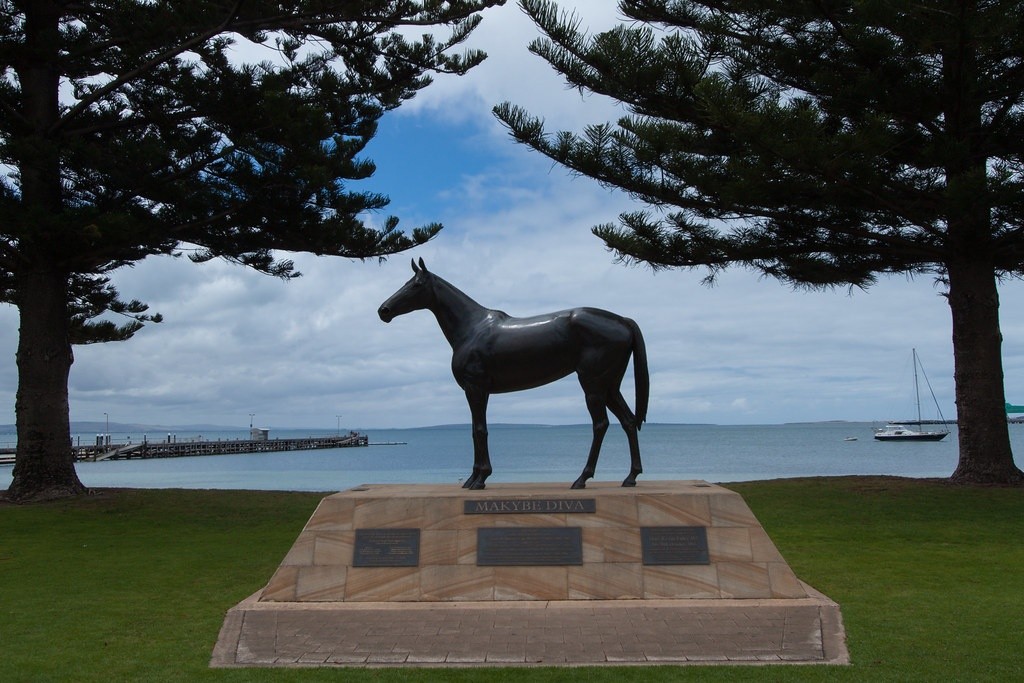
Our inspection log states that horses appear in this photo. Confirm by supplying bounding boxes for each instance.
[378,257,649,491]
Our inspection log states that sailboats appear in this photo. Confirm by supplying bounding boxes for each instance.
[872,347,949,442]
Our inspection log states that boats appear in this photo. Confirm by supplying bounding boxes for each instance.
[842,434,858,442]
